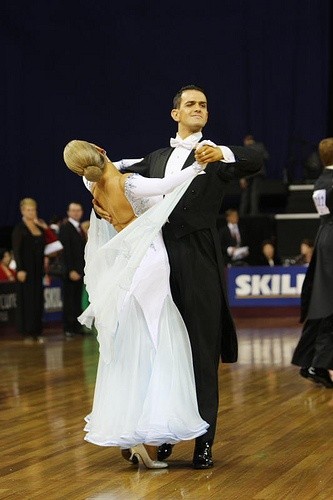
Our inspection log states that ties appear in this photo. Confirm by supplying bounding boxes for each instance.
[79,225,86,239]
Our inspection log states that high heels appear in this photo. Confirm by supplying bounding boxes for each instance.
[120,448,138,463]
[128,444,168,469]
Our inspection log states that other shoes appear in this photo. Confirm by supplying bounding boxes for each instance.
[308,367,333,388]
[300,368,318,383]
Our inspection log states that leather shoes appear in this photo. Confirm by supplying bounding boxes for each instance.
[192,442,213,468]
[157,443,172,461]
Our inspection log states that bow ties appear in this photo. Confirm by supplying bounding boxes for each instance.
[170,137,195,150]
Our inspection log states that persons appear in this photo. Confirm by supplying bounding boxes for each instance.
[290,138,333,389]
[12,198,49,344]
[63,140,218,469]
[0,134,315,282]
[59,201,90,337]
[91,85,265,469]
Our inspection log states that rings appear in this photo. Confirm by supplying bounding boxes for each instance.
[202,152,205,155]
